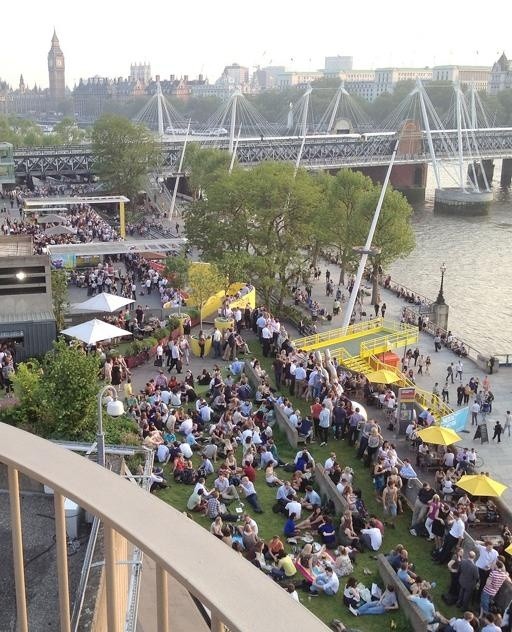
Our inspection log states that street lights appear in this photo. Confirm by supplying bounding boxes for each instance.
[435,262,448,306]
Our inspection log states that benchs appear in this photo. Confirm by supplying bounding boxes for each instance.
[374,550,438,632]
[273,401,306,449]
[243,356,262,393]
[312,462,359,521]
[231,330,246,358]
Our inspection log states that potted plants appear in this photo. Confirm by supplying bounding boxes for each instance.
[211,254,250,336]
[184,261,227,358]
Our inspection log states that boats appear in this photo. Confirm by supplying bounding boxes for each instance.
[34,120,232,137]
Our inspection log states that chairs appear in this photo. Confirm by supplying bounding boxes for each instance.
[368,387,512,578]
